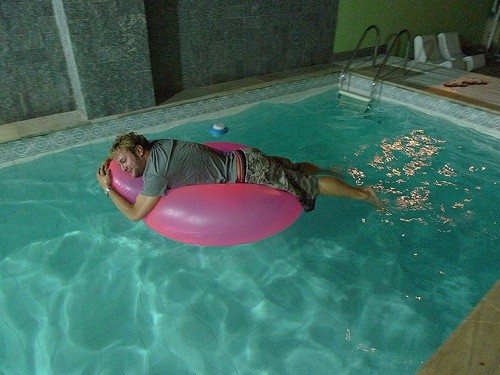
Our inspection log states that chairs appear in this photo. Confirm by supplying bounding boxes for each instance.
[414,34,467,72]
[437,32,485,72]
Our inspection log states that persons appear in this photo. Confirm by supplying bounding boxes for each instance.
[95,132,389,223]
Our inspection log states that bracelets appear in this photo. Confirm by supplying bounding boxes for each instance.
[104,186,112,197]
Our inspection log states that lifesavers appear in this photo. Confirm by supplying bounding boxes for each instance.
[106,141,304,244]
